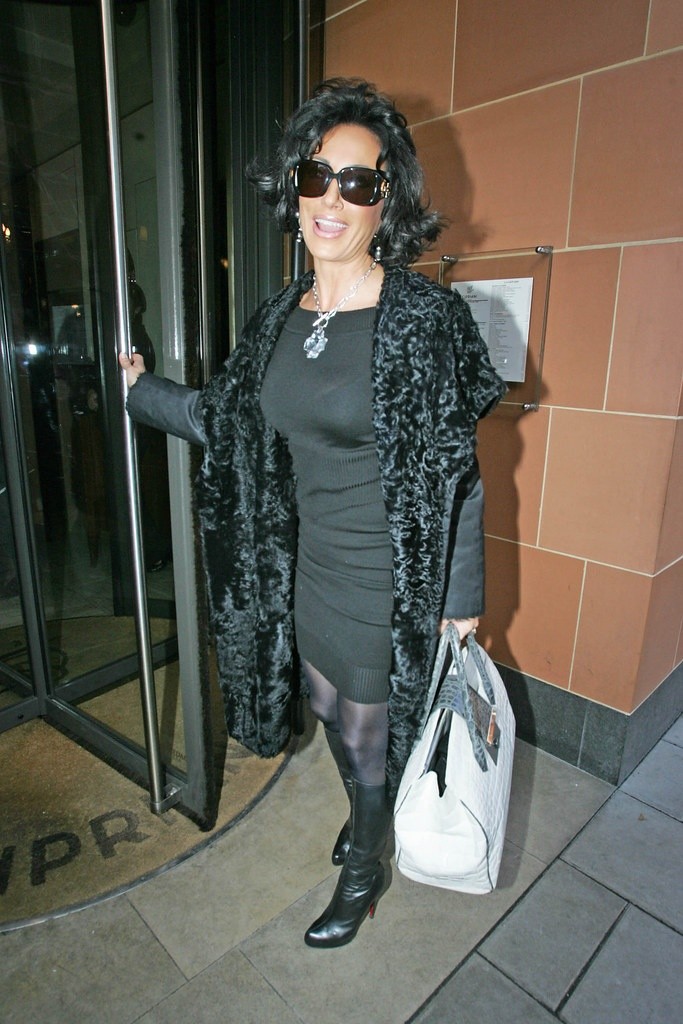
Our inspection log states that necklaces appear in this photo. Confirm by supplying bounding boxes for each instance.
[303,262,376,360]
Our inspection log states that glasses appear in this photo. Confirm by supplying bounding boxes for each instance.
[290,158,393,206]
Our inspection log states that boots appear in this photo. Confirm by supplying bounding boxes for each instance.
[323,725,357,867]
[304,775,389,949]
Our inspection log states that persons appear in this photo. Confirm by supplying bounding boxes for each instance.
[117,77,504,948]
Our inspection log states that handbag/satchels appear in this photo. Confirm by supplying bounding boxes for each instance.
[392,621,516,895]
